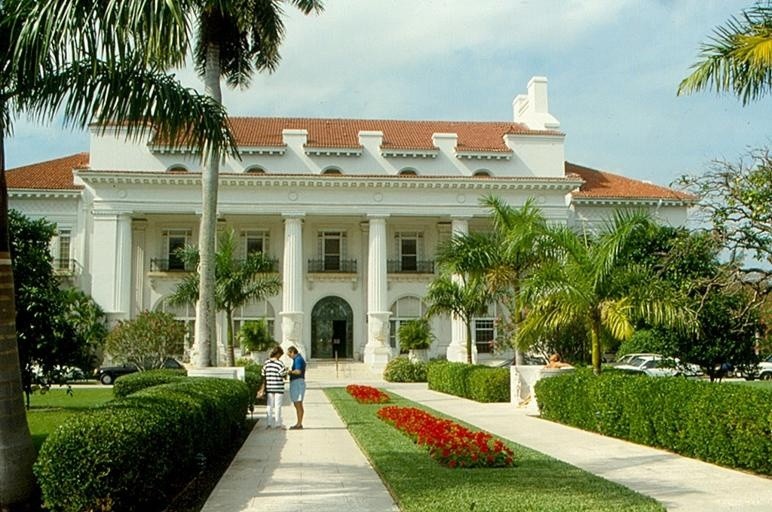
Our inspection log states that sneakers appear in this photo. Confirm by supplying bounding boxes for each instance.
[290,425,303,430]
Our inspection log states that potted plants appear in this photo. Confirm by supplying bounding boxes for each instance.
[238,315,281,365]
[398,319,437,365]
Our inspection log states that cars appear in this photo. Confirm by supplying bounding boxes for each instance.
[604,350,701,380]
[703,350,755,378]
[29,359,86,385]
[91,355,188,386]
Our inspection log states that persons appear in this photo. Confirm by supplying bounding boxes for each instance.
[287,346,306,429]
[517,353,570,407]
[261,346,289,431]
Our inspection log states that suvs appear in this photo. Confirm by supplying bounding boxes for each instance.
[740,353,772,383]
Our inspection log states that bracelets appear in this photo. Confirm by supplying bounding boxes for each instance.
[291,372,292,375]
